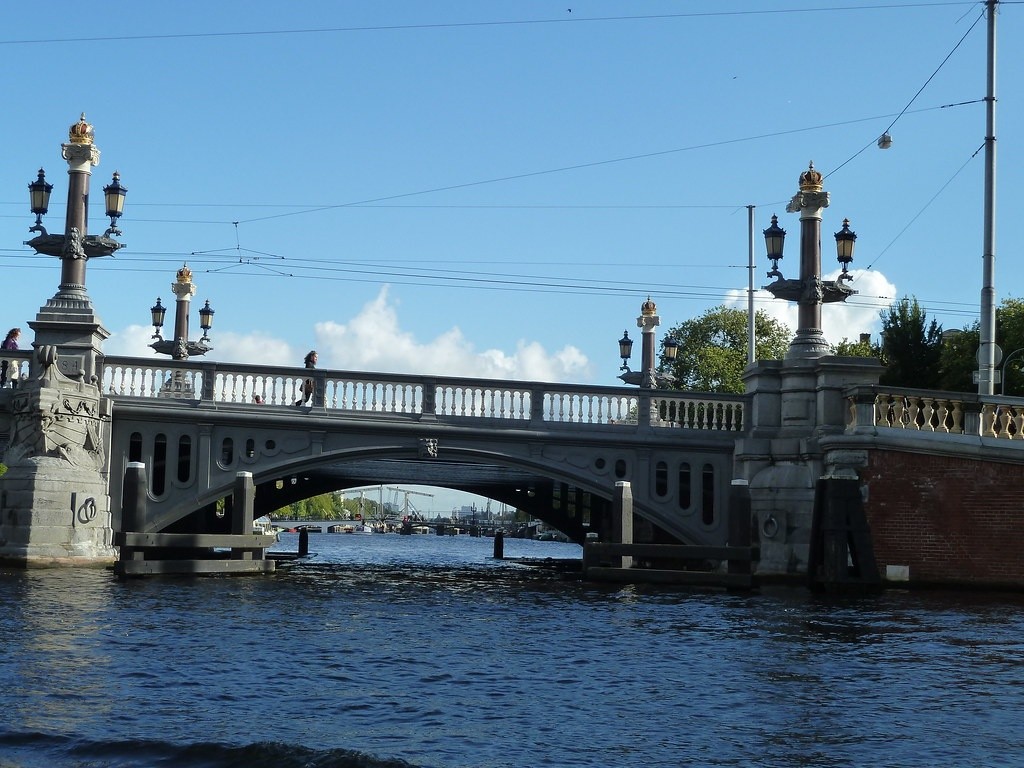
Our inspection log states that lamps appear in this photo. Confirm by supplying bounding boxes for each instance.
[762,214,787,271]
[150,296,167,335]
[103,169,128,231]
[878,134,892,148]
[28,167,54,226]
[834,219,857,275]
[198,299,215,338]
[664,336,679,365]
[618,330,633,367]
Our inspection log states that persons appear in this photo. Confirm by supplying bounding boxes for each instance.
[290,351,318,407]
[0,328,20,388]
[255,395,262,406]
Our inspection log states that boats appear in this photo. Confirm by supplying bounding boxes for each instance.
[253,516,280,542]
[327,524,354,533]
[294,525,322,533]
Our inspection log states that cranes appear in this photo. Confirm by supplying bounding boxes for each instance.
[386,487,434,505]
[341,487,383,514]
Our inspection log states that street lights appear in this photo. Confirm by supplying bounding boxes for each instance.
[147,262,215,376]
[616,294,678,406]
[761,160,859,336]
[22,113,127,290]
